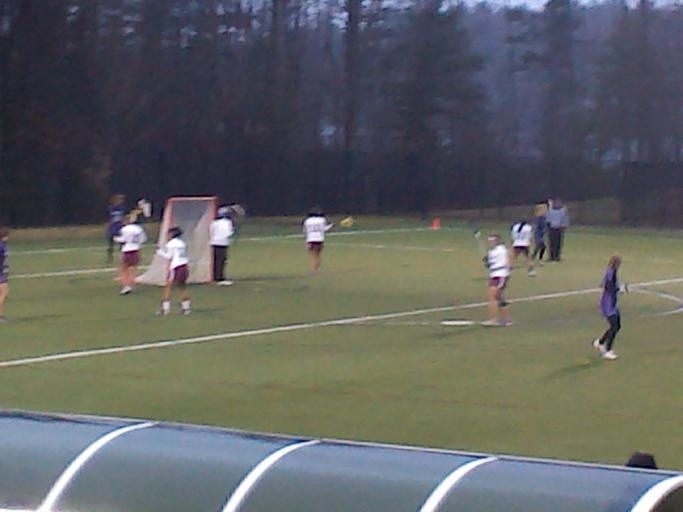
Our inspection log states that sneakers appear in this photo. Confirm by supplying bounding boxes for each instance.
[214,278,236,287]
[119,285,134,296]
[592,338,620,361]
[478,316,517,330]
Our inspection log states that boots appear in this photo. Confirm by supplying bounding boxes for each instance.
[177,297,193,317]
[153,298,173,317]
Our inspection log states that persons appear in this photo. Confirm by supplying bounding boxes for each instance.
[591,255,623,360]
[301,210,335,271]
[105,193,244,317]
[0,230,11,322]
[481,196,569,327]
